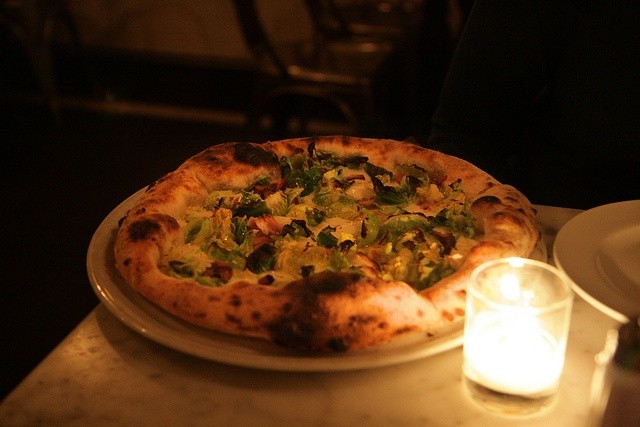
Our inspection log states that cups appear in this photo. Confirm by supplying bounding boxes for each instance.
[463,258,573,421]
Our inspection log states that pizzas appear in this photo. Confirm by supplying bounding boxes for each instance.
[112,132,540,355]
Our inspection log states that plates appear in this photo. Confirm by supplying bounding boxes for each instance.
[553,200,640,323]
[88,180,547,374]
